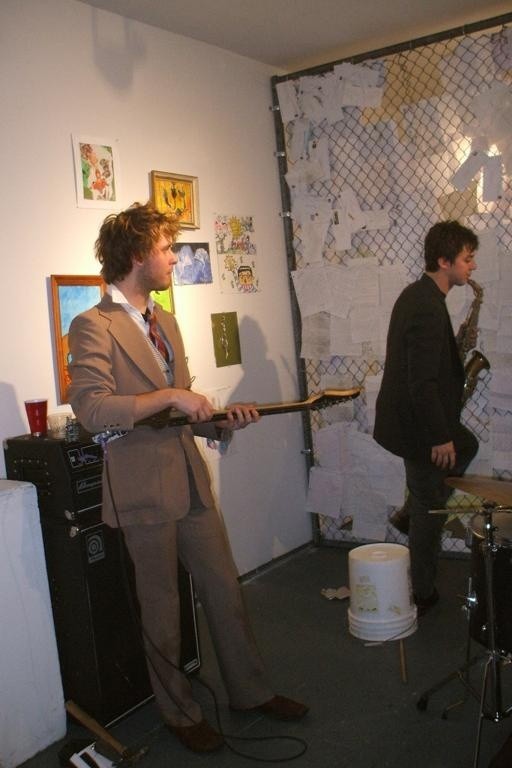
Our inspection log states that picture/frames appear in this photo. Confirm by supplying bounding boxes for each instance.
[150,169,199,231]
[50,273,107,401]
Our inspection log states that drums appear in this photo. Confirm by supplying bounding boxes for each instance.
[468,513,512,662]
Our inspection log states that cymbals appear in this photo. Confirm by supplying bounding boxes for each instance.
[445,476,511,506]
[427,508,512,515]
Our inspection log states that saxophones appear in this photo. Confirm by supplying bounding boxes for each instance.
[456,277,489,411]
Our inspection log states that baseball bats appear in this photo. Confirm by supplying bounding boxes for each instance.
[64,690,131,758]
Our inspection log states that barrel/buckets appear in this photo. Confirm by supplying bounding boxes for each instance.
[347,541,419,647]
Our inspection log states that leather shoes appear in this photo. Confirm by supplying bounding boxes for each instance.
[164,718,225,753]
[412,587,438,606]
[388,509,409,535]
[231,694,311,719]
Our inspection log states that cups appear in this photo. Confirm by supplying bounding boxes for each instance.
[24,399,48,437]
[48,413,67,438]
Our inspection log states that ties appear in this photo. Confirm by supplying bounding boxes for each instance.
[145,313,168,362]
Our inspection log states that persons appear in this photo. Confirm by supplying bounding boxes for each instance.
[67,203,312,755]
[371,219,479,617]
